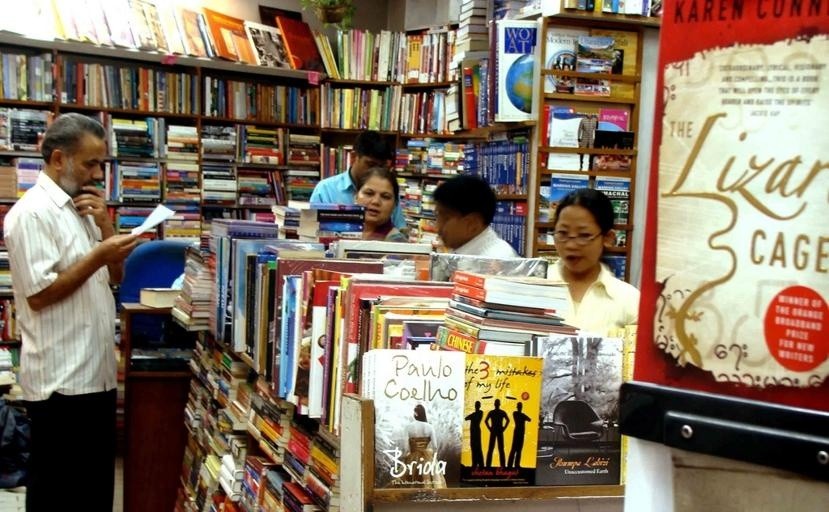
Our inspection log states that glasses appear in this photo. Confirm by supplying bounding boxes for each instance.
[551,231,602,246]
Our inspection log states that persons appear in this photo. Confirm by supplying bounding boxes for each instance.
[309,129,408,236]
[353,169,409,246]
[3,112,143,512]
[543,187,643,340]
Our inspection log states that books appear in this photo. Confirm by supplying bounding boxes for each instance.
[362,349,466,490]
[429,269,580,355]
[544,28,637,99]
[320,84,444,134]
[446,0,537,133]
[140,288,181,308]
[564,0,661,18]
[1,0,329,75]
[320,144,355,182]
[397,177,527,262]
[1,44,326,126]
[3,108,53,217]
[536,182,630,282]
[94,113,319,248]
[171,429,341,512]
[171,201,455,435]
[312,28,454,82]
[0,246,21,401]
[397,137,530,195]
[525,337,619,486]
[540,105,631,172]
[460,357,543,488]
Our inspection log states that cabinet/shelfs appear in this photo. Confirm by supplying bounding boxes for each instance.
[1,3,659,511]
